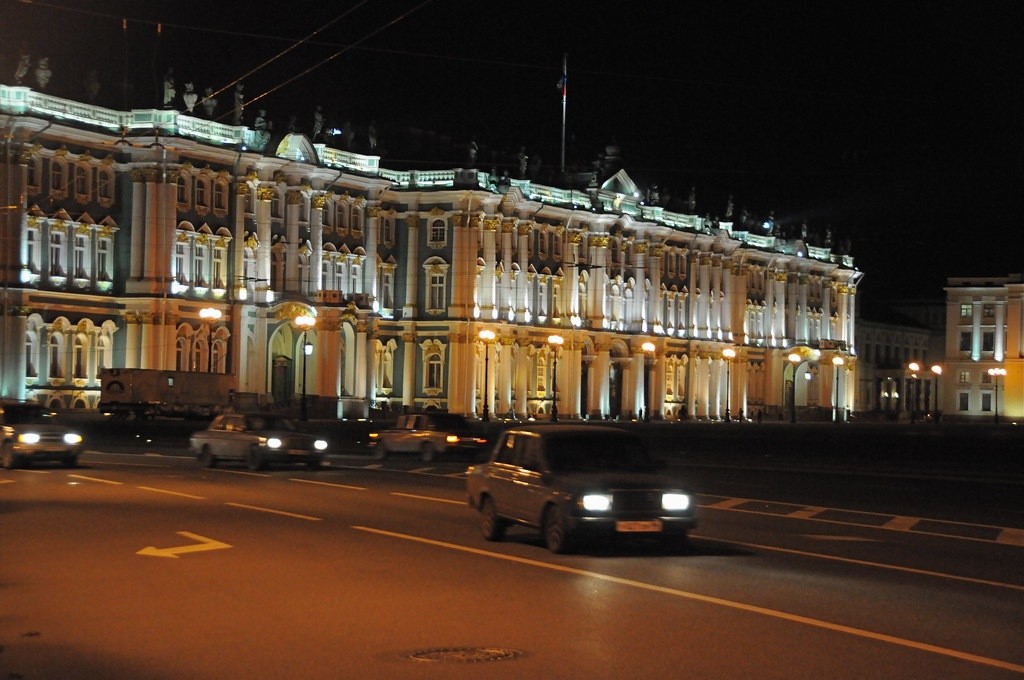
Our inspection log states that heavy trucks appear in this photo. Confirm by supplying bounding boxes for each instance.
[97,364,268,428]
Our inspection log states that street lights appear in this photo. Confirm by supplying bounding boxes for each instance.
[988,368,1007,423]
[932,365,943,422]
[909,362,921,425]
[721,348,736,424]
[295,316,316,418]
[641,342,656,421]
[479,329,495,422]
[831,357,846,423]
[786,354,802,422]
[549,334,564,419]
[200,307,220,374]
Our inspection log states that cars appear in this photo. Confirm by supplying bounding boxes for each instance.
[0,396,85,469]
[187,413,332,471]
[463,424,701,556]
[369,411,491,464]
